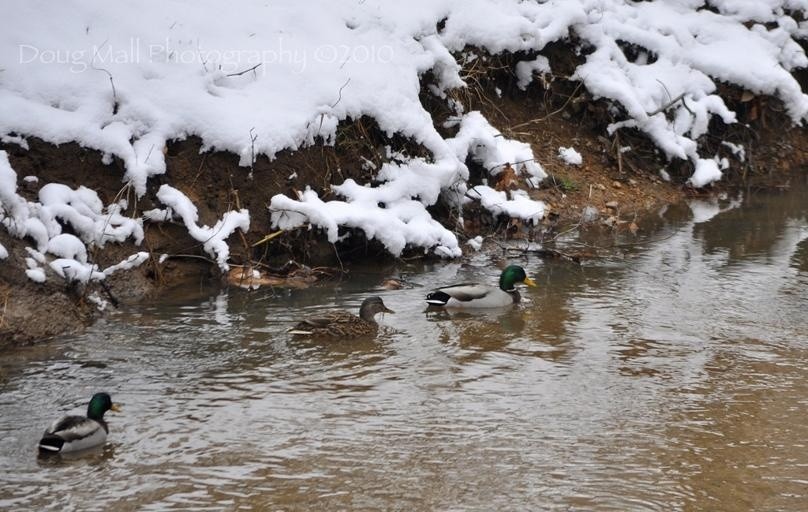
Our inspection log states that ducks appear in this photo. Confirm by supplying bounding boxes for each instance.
[38,393,122,455]
[425,265,537,306]
[285,297,395,338]
